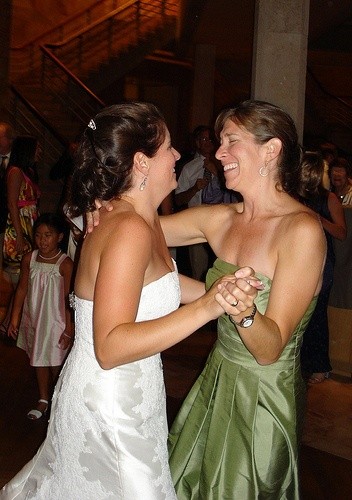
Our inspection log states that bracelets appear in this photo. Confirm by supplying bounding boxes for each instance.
[64,332,71,338]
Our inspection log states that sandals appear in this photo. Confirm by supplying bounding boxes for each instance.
[26,399,49,421]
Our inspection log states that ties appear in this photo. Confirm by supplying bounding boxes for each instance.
[203,169,213,182]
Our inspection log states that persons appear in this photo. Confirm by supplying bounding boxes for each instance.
[0,102,261,500]
[327,157,352,377]
[50,136,83,215]
[0,123,16,180]
[0,135,42,340]
[320,144,337,189]
[289,153,346,383]
[86,99,327,500]
[174,125,243,209]
[7,213,76,420]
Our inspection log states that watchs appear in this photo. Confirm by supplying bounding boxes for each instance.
[230,304,257,328]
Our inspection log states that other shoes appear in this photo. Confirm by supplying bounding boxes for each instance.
[308,372,330,384]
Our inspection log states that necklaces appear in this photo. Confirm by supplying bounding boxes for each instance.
[39,249,62,260]
